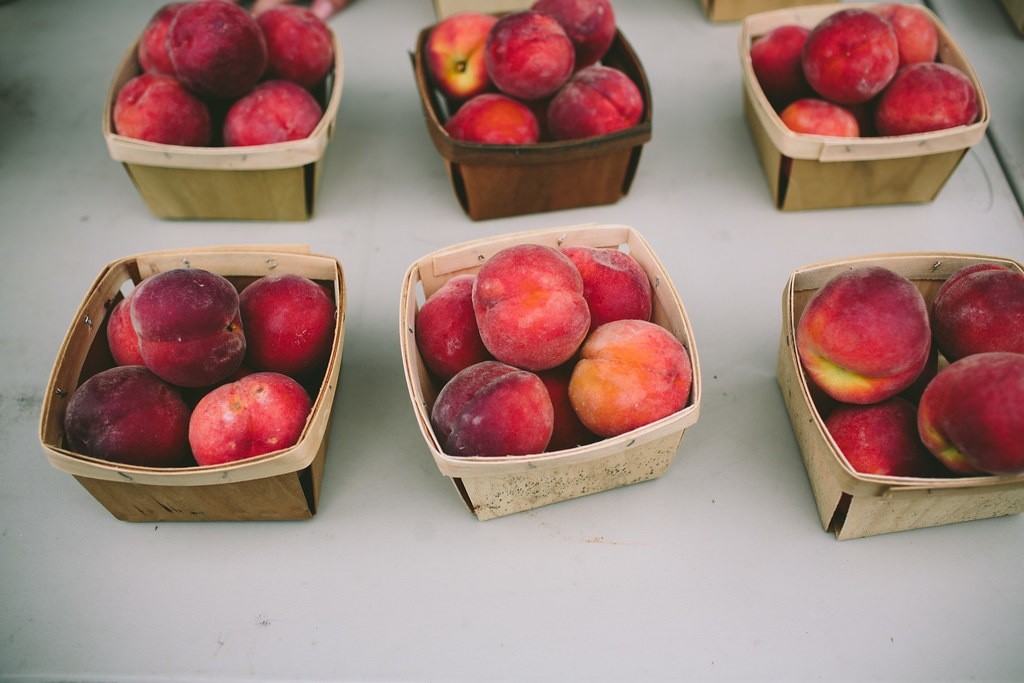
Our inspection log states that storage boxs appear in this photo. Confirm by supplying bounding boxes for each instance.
[37,242,347,524]
[101,0,343,222]
[398,222,703,524]
[736,0,991,215]
[776,251,1024,541]
[407,6,655,225]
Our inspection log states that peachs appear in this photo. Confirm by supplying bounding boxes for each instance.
[62,1,1024,485]
